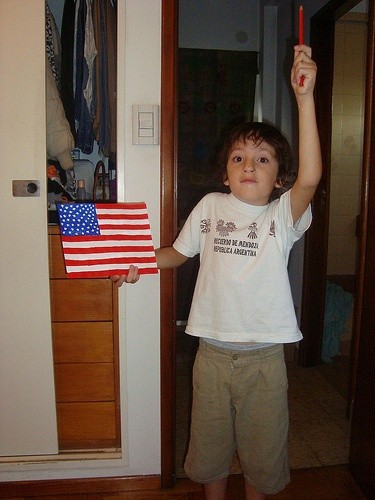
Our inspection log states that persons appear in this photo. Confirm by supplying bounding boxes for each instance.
[111,44,322,500]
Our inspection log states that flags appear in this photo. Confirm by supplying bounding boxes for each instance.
[57,203,159,278]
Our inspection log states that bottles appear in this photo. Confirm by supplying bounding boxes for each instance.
[77,180,86,200]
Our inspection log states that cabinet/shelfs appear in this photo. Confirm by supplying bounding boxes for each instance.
[48,226,121,449]
